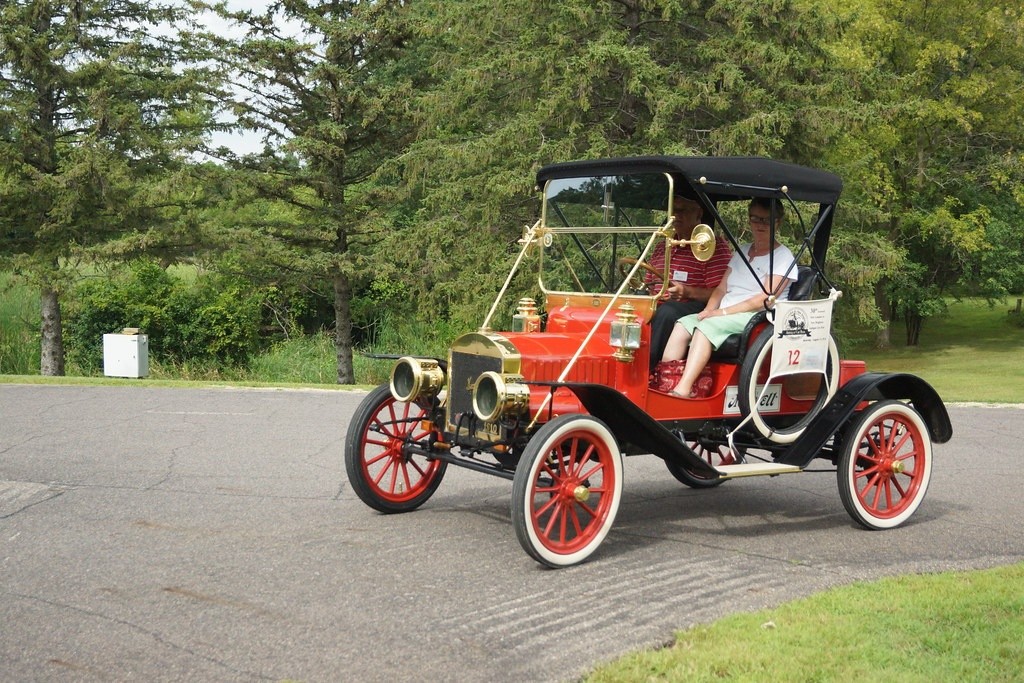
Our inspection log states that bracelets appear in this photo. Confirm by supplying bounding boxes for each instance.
[722,308,727,316]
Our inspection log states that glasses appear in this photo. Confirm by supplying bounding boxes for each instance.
[750,214,778,224]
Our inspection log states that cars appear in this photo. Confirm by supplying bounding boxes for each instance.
[346,154,954,571]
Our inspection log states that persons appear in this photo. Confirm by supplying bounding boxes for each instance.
[643,188,800,399]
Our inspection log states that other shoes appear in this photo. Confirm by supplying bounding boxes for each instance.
[668,390,690,401]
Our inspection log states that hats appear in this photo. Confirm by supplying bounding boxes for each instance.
[661,186,703,205]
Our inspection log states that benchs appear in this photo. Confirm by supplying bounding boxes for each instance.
[686,264,818,363]
